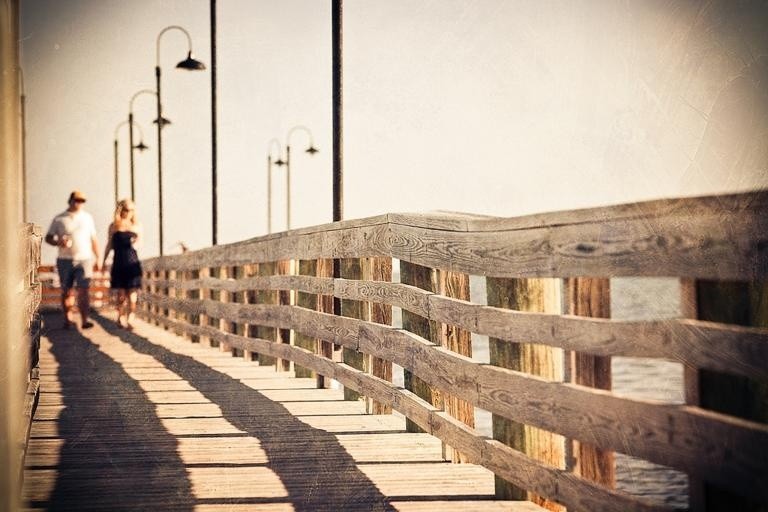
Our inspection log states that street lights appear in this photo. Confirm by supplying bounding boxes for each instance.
[264,124,318,231]
[154,23,207,260]
[113,89,169,212]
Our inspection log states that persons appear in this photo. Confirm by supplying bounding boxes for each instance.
[101,200,143,330]
[45,192,100,329]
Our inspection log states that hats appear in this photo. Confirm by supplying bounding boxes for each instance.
[70,192,85,202]
